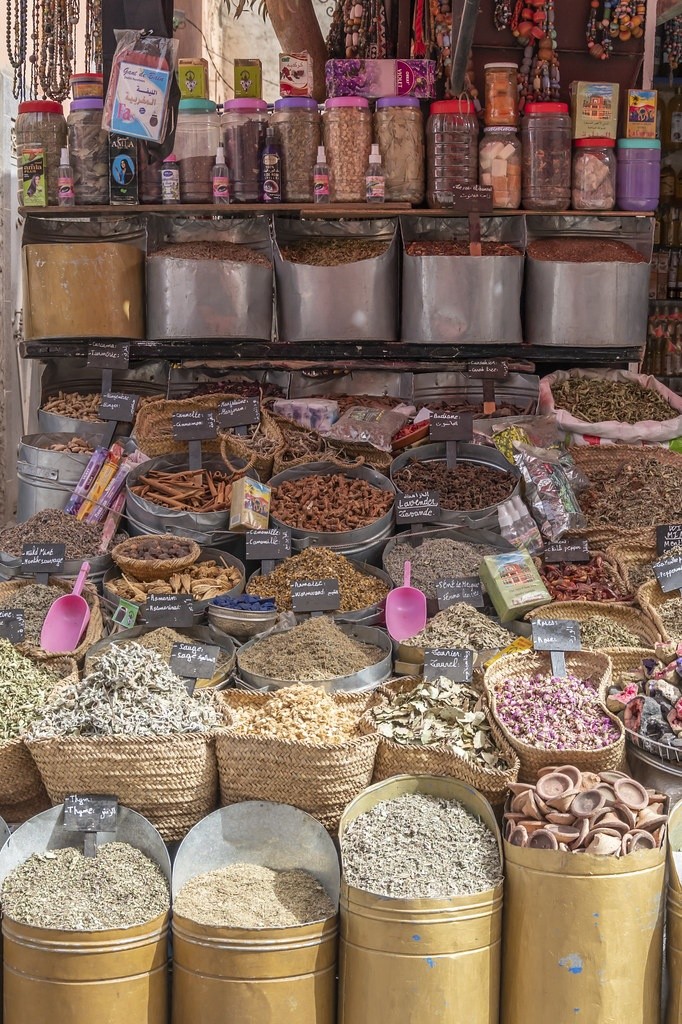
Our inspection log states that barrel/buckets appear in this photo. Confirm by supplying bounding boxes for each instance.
[0,216,656,699]
[0,773,681,1024]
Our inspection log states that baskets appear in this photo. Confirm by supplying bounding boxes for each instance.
[1,394,682,871]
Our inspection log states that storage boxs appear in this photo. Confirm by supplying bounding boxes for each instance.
[278,50,316,98]
[272,215,399,342]
[401,213,527,344]
[528,215,657,348]
[324,57,436,99]
[568,79,620,141]
[177,57,209,100]
[19,212,145,341]
[479,546,552,625]
[622,88,658,140]
[147,213,272,342]
[22,147,48,208]
[107,131,140,206]
[233,58,264,99]
[229,476,271,533]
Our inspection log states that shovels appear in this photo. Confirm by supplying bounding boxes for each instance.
[41,562,91,650]
[385,561,427,642]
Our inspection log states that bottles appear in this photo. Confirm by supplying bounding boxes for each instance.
[211,142,231,205]
[373,96,424,205]
[425,100,478,209]
[273,97,322,203]
[365,144,385,203]
[574,138,617,212]
[311,145,331,204]
[617,140,661,212]
[642,24,681,396]
[56,146,75,207]
[261,127,283,204]
[68,73,103,99]
[322,98,373,202]
[478,126,523,210]
[15,101,67,206]
[160,155,181,204]
[483,62,517,128]
[170,98,220,203]
[521,102,573,211]
[67,99,111,205]
[220,98,268,202]
